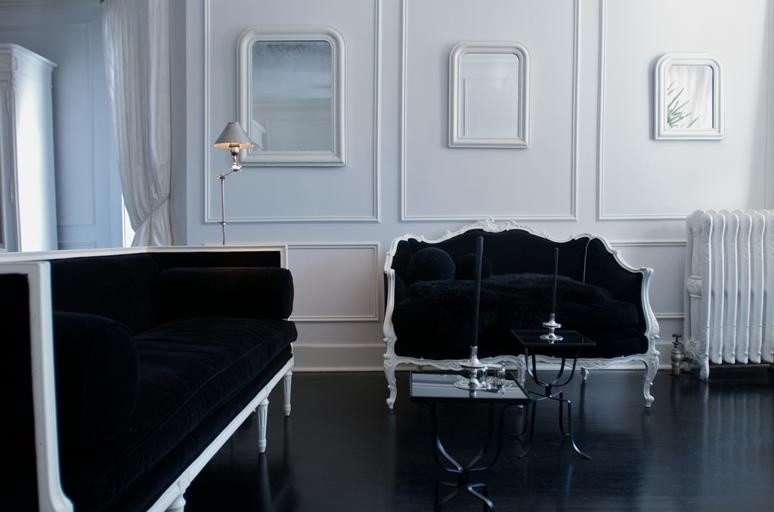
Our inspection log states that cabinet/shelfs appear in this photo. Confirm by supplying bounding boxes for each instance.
[0,43,59,253]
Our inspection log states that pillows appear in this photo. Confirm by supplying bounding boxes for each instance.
[400,247,456,284]
[53,310,141,421]
[152,267,294,321]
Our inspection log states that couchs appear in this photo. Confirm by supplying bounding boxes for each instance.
[392,219,661,411]
[0,245,298,512]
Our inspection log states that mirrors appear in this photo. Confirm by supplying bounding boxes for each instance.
[448,41,529,149]
[652,52,723,141]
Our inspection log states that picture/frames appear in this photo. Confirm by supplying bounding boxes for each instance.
[235,26,345,167]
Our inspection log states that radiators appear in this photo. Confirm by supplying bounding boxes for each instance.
[683,208,774,382]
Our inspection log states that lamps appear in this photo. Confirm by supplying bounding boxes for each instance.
[212,121,255,245]
